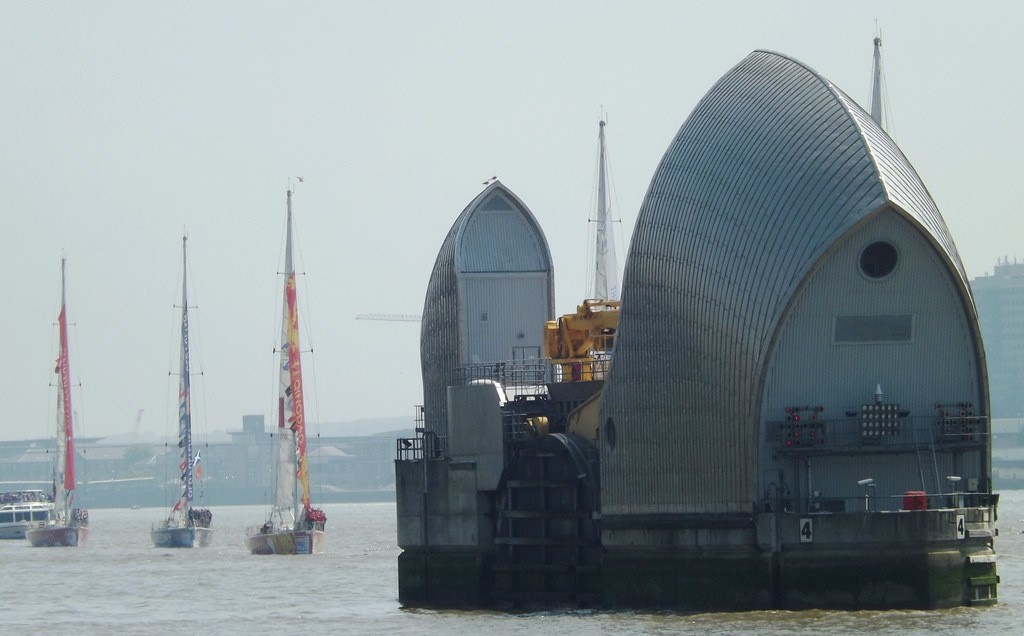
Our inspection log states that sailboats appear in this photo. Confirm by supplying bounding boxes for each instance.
[148,235,216,550]
[240,177,330,557]
[24,256,91,548]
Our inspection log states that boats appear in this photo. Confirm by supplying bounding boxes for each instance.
[389,24,1006,611]
[0,487,59,542]
